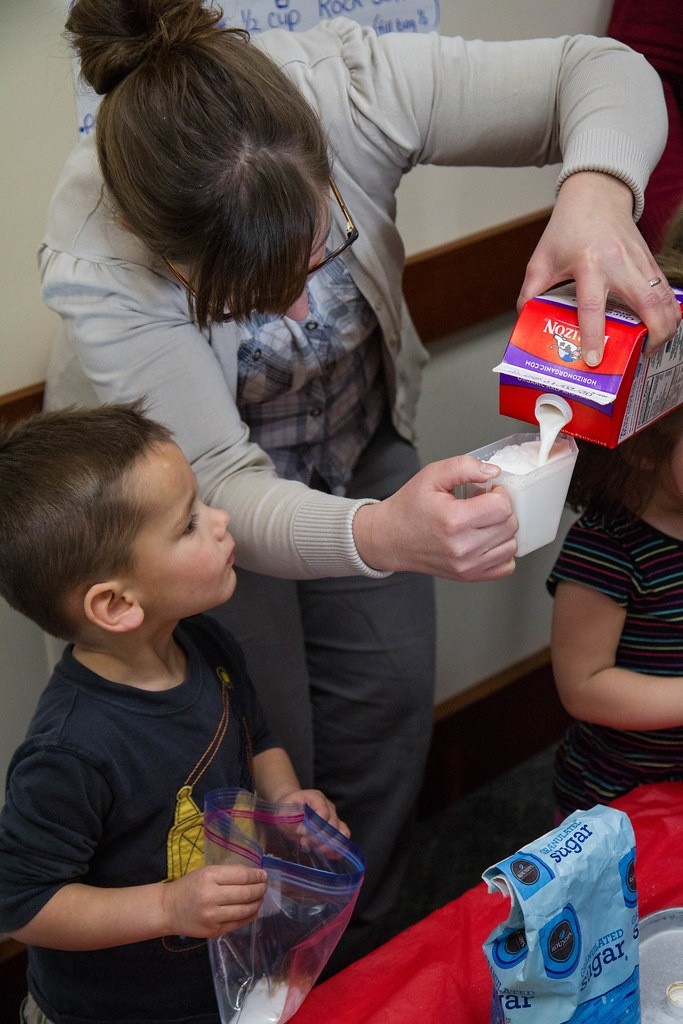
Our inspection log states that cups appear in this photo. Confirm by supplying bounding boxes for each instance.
[460,432,579,558]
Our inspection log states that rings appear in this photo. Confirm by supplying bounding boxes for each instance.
[647,274,663,288]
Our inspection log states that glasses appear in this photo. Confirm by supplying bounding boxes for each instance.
[161,175,359,324]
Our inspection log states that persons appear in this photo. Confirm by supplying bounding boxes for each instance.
[0,389,351,1023]
[545,405,683,829]
[31,0,672,960]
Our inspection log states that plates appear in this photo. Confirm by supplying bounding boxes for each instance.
[639,908,683,1024]
